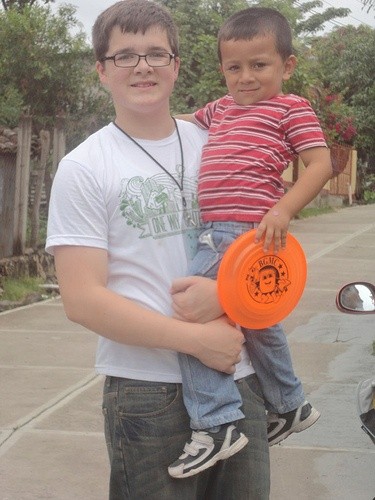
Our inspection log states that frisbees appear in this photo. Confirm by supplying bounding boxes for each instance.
[216,226,307,330]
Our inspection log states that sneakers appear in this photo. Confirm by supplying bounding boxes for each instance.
[168,423,249,479]
[266,401,321,447]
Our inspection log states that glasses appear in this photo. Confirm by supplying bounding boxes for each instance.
[98,51,175,68]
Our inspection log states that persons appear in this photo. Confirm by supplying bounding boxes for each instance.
[167,7,334,478]
[46,0,270,499]
